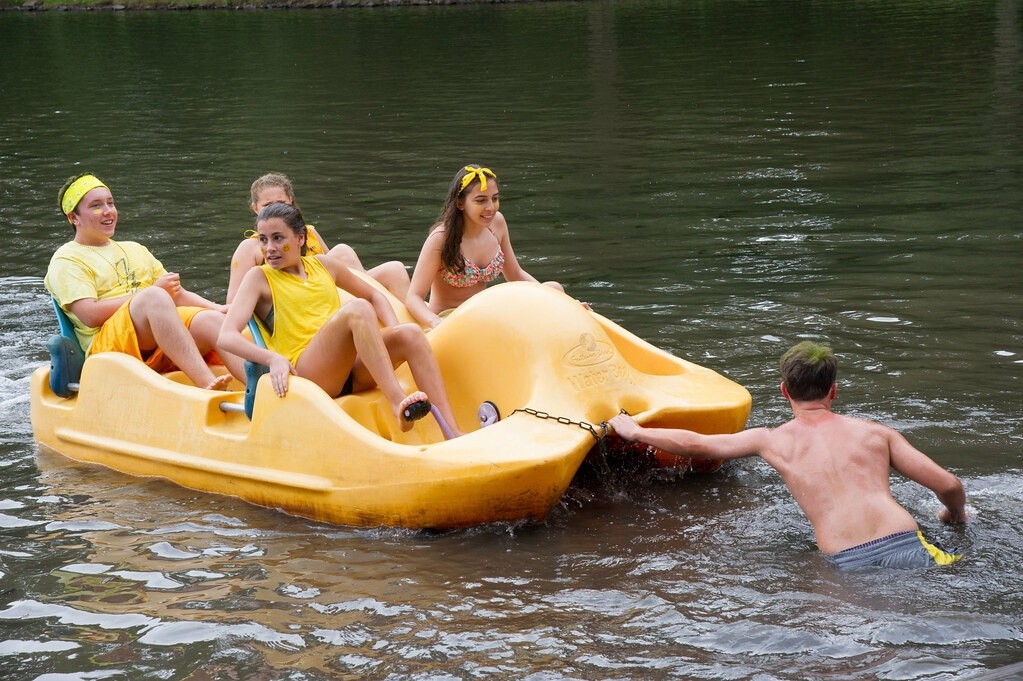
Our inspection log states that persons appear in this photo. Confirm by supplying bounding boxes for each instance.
[227,173,447,329]
[216,203,467,440]
[405,164,593,329]
[44,173,248,389]
[608,340,965,573]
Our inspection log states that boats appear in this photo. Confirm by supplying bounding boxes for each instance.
[30,281,752,528]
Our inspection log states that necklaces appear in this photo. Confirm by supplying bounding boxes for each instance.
[80,238,130,279]
